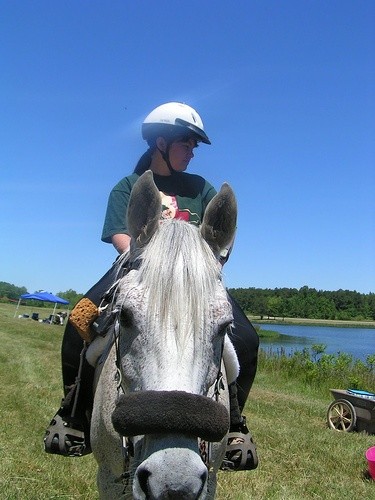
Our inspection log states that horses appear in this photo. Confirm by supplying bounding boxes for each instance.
[87,170,240,500]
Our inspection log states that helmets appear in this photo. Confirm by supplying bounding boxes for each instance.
[142,101,212,146]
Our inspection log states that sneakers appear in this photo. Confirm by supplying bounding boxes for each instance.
[229,425,252,462]
[45,414,89,447]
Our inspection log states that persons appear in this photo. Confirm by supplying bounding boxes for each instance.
[43,102,260,469]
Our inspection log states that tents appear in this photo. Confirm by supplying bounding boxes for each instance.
[14,292,69,327]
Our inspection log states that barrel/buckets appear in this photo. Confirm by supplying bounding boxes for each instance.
[366,446,375,479]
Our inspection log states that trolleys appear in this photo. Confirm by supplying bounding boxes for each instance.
[326,388,375,433]
